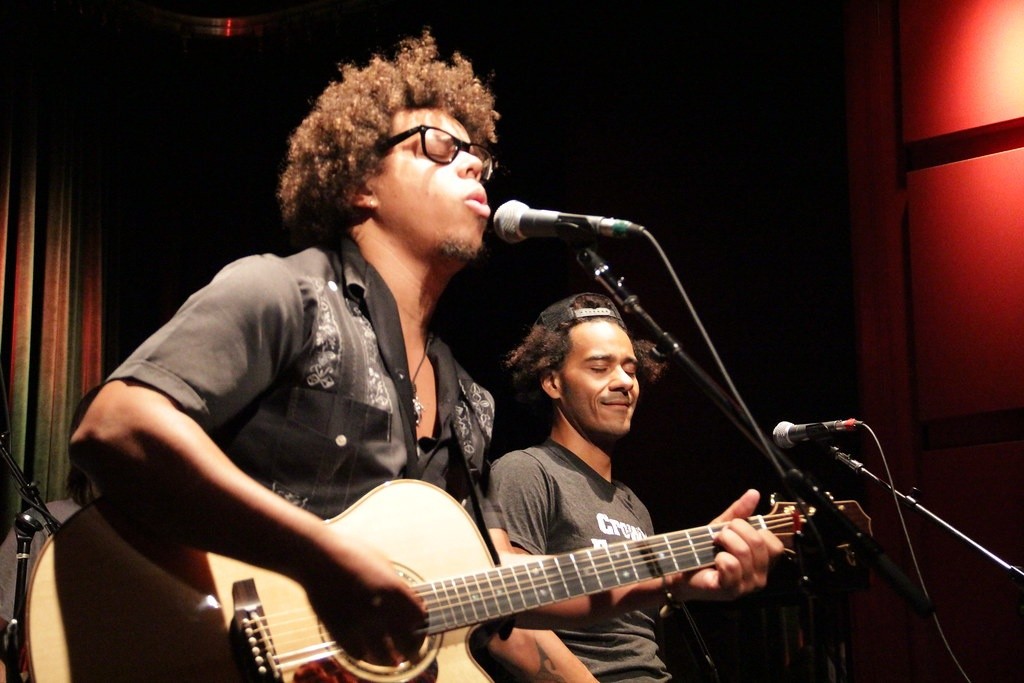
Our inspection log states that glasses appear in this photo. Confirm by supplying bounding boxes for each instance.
[380,124,498,180]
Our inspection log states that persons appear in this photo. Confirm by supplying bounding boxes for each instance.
[491,290,678,683]
[67,27,784,682]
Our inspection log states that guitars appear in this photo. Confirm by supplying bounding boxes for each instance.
[15,476,876,683]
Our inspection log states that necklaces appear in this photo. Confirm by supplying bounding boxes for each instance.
[406,329,433,424]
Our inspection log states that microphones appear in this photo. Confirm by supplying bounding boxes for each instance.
[772,418,862,449]
[493,198,647,245]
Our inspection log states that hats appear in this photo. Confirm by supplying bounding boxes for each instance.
[535,294,628,335]
